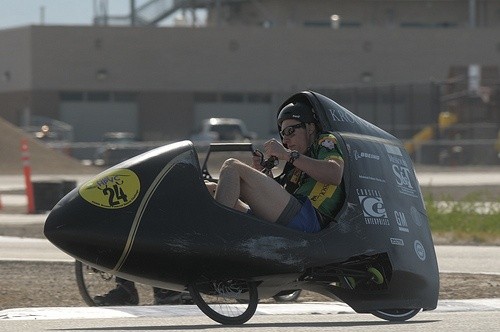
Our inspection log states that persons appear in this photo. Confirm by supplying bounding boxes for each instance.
[203,100,348,231]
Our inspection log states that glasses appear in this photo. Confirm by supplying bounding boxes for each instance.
[279,123,304,138]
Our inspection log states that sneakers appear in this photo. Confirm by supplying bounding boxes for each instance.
[152,288,194,305]
[95,283,139,305]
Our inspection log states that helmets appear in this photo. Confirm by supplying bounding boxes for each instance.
[278,102,318,128]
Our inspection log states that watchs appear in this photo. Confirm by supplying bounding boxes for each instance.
[288,150,299,165]
